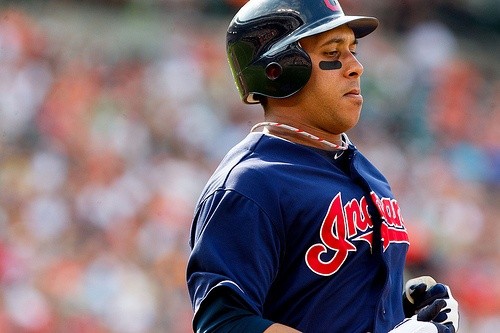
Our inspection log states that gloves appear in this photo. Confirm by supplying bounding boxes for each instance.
[385,299,457,333]
[402,274,460,333]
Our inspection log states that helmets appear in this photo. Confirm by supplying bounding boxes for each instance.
[223,0,380,105]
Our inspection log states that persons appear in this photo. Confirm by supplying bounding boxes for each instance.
[187,0,461,333]
